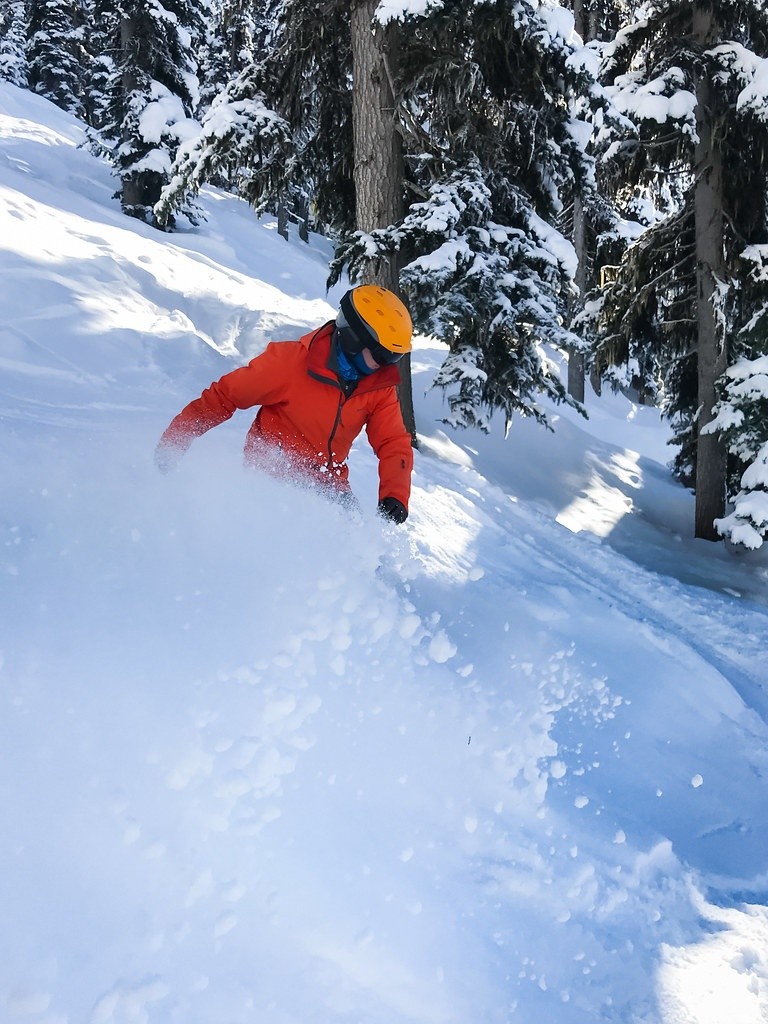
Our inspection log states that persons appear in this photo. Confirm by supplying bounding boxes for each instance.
[158,284,414,524]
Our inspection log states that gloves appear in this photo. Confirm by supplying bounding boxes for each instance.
[378,497,408,525]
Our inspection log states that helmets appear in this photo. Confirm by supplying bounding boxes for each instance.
[334,285,412,353]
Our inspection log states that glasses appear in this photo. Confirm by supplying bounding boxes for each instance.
[370,343,405,365]
